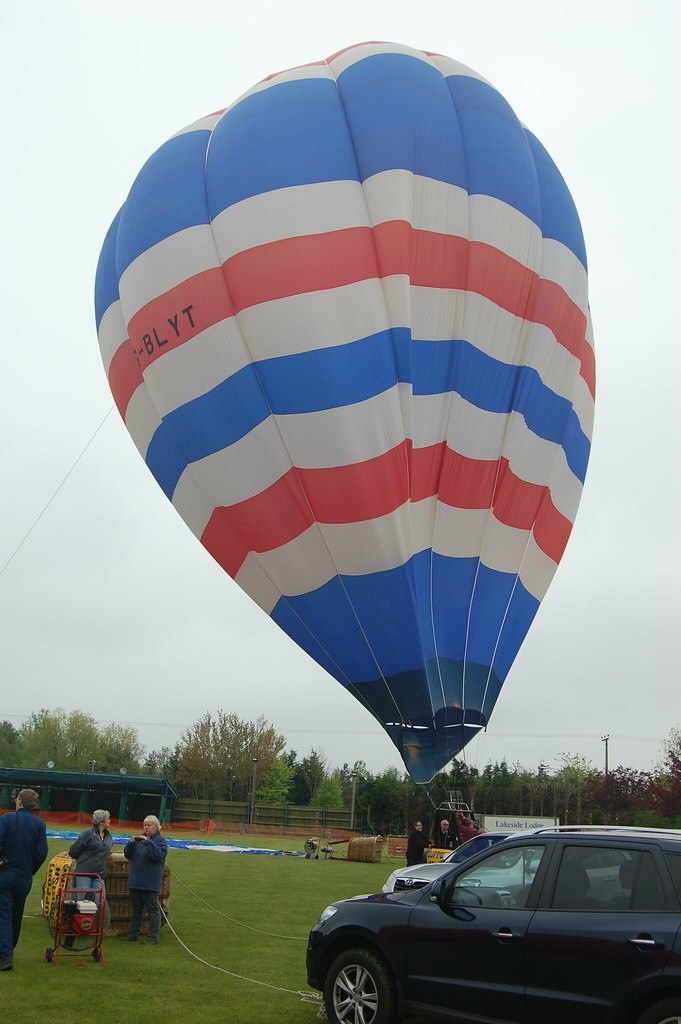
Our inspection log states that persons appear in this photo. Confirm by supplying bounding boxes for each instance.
[405,821,435,868]
[68,809,113,904]
[435,811,479,850]
[0,788,49,973]
[120,815,168,946]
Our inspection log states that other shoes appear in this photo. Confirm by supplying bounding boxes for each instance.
[140,938,159,944]
[121,935,139,942]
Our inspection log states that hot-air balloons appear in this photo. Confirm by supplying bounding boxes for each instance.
[93,38,600,865]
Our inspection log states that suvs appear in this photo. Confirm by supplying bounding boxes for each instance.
[304,823,681,1024]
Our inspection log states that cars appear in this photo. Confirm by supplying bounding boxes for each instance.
[381,830,635,904]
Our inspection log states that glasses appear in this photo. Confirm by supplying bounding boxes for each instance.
[441,823,449,825]
[415,823,423,827]
[15,799,20,802]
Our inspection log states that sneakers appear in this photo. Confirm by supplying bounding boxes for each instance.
[0,961,13,971]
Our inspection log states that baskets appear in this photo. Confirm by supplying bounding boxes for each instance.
[347,834,383,863]
[387,835,408,857]
[103,851,170,937]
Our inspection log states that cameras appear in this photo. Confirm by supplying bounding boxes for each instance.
[135,836,142,841]
